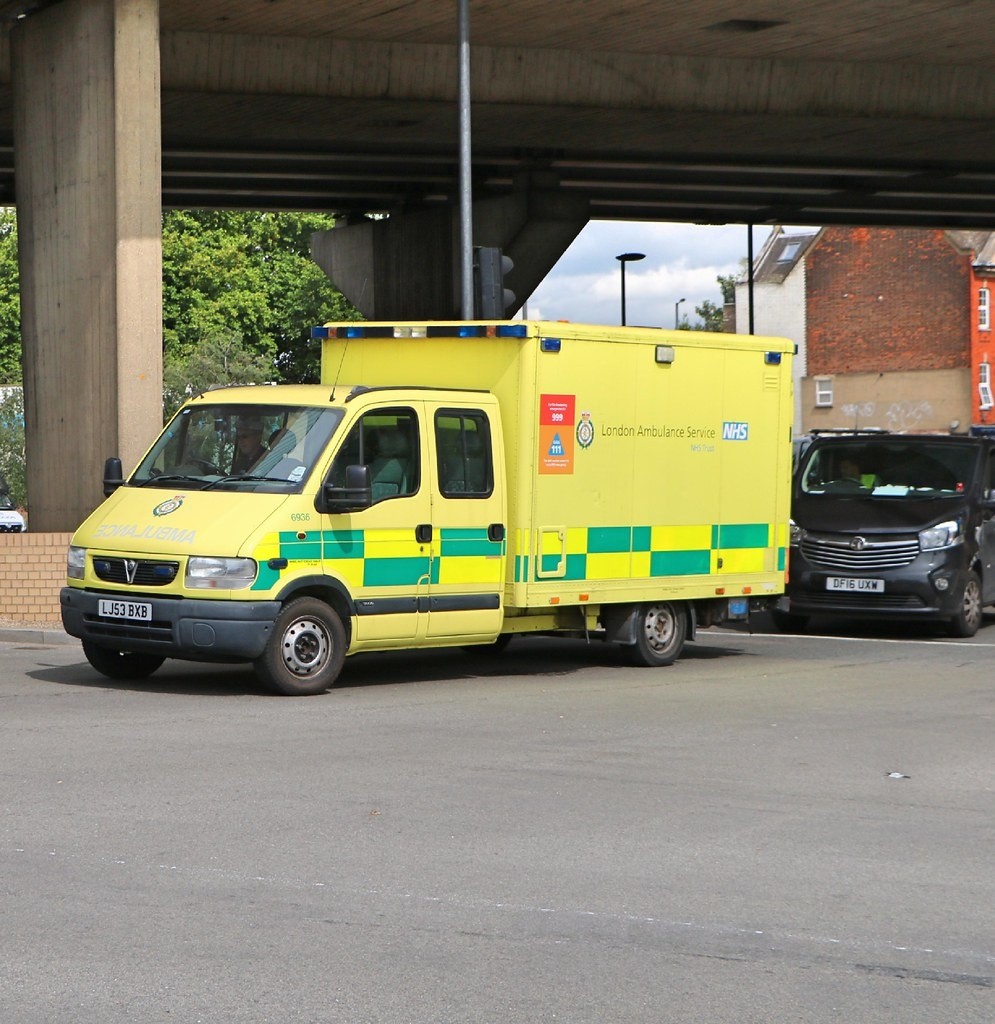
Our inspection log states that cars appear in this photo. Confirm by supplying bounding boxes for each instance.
[0,487,26,534]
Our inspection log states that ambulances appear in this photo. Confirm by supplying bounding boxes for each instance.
[57,317,800,699]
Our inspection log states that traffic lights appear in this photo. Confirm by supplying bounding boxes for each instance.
[473,244,516,317]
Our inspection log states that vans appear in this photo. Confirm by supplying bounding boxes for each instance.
[788,420,995,638]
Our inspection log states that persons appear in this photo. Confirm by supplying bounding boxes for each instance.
[231,420,278,479]
[840,458,884,491]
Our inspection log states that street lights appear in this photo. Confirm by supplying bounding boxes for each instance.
[615,252,647,326]
[675,298,685,329]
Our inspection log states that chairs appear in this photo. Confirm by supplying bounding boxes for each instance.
[367,427,416,504]
[444,432,485,491]
[265,429,304,480]
